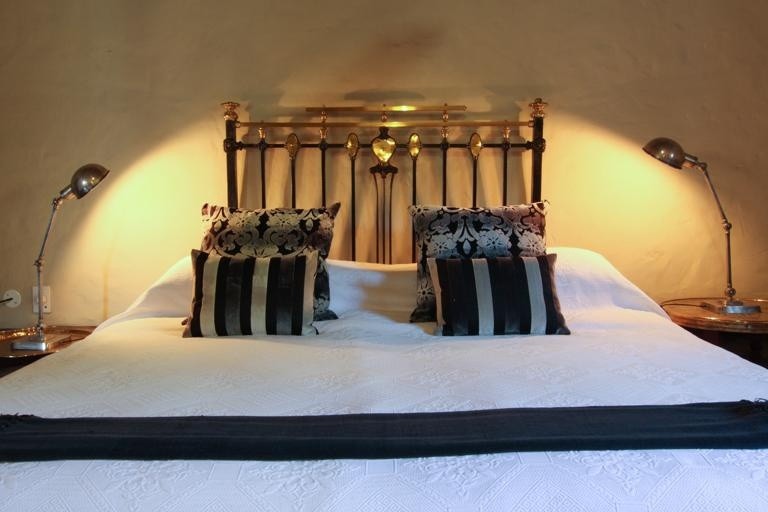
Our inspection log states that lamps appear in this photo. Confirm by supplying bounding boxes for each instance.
[638,136,763,316]
[12,163,112,349]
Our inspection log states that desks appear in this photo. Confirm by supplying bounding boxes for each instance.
[1,325,92,367]
[661,294,767,364]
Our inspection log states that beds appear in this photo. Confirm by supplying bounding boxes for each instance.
[0,98,766,512]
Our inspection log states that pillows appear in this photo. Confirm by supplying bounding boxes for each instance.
[195,198,345,324]
[182,245,324,340]
[425,254,572,338]
[405,198,546,324]
